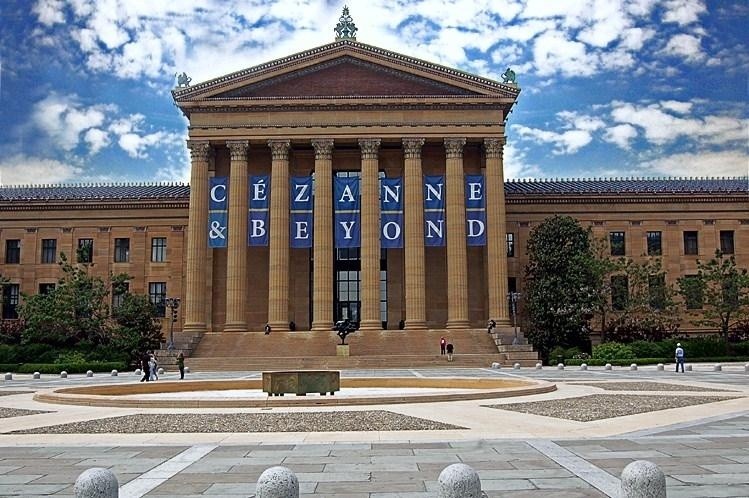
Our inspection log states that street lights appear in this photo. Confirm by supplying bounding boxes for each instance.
[506,291,523,345]
[165,297,180,350]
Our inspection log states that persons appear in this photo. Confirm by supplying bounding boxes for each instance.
[446,342,455,361]
[488,319,497,333]
[264,324,271,334]
[176,352,184,379]
[675,344,686,372]
[139,350,159,381]
[440,337,447,355]
